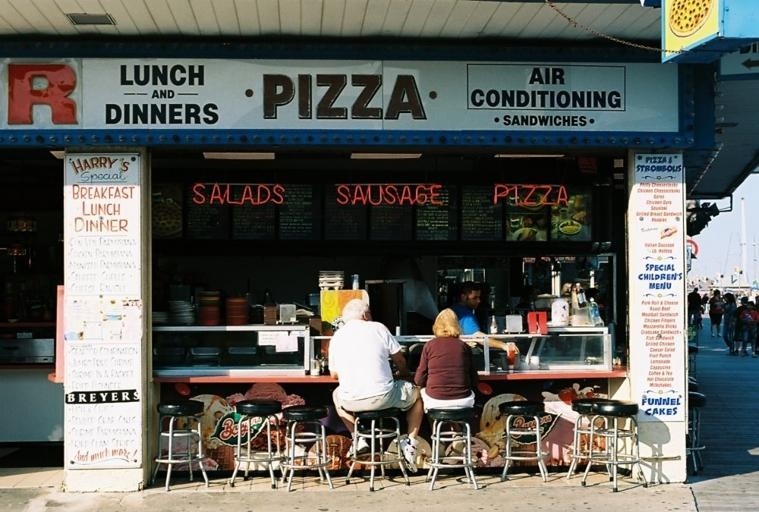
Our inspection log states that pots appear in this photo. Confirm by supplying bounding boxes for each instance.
[155,342,188,366]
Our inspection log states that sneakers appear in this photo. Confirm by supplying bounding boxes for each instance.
[345,435,370,461]
[393,434,418,474]
[717,334,721,337]
[439,436,466,465]
[729,350,758,358]
[711,333,715,337]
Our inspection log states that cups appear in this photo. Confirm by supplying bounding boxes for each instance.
[3,295,16,323]
[310,358,328,376]
[250,304,265,324]
[498,353,508,368]
[506,343,516,364]
[559,209,568,219]
[529,356,539,369]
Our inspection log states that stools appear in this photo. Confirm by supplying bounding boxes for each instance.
[148,399,211,492]
[579,401,649,493]
[340,406,411,493]
[495,400,550,484]
[228,398,290,490]
[687,340,708,476]
[421,408,479,491]
[277,403,335,493]
[566,397,623,483]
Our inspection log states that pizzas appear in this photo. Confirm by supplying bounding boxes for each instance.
[668,0,711,37]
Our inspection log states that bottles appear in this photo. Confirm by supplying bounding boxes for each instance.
[490,315,497,334]
[352,274,359,290]
[589,297,601,325]
[575,282,587,307]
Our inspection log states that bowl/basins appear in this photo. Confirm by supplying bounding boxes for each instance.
[259,344,299,364]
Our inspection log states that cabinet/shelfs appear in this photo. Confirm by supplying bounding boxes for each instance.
[309,333,495,378]
[150,322,311,379]
[482,333,617,375]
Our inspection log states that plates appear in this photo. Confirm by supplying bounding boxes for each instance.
[225,297,250,323]
[152,299,196,327]
[511,228,537,242]
[559,220,581,235]
[196,290,222,325]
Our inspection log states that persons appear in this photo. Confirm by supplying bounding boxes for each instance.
[687,287,759,358]
[415,307,476,457]
[452,281,520,357]
[328,299,425,474]
